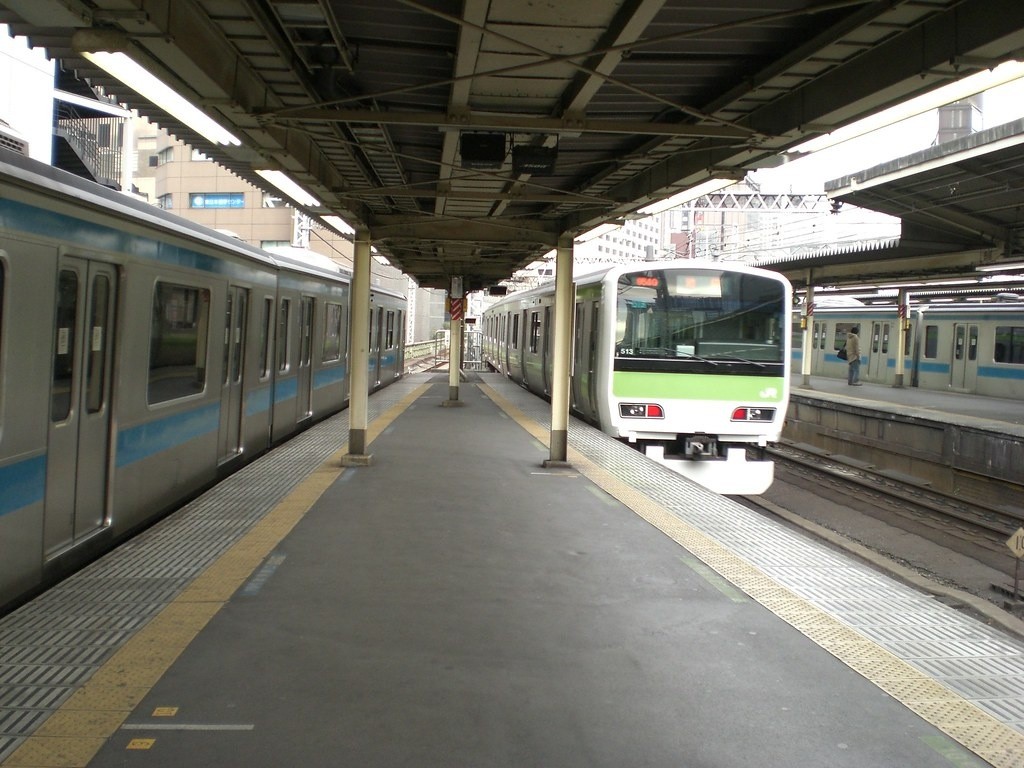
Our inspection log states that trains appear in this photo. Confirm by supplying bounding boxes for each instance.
[1,143,409,611]
[479,262,799,496]
[785,302,1024,404]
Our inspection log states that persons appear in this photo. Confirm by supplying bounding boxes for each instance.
[846,327,863,386]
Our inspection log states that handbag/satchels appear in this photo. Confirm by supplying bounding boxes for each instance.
[836,349,848,360]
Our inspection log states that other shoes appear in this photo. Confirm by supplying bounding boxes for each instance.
[849,381,862,386]
